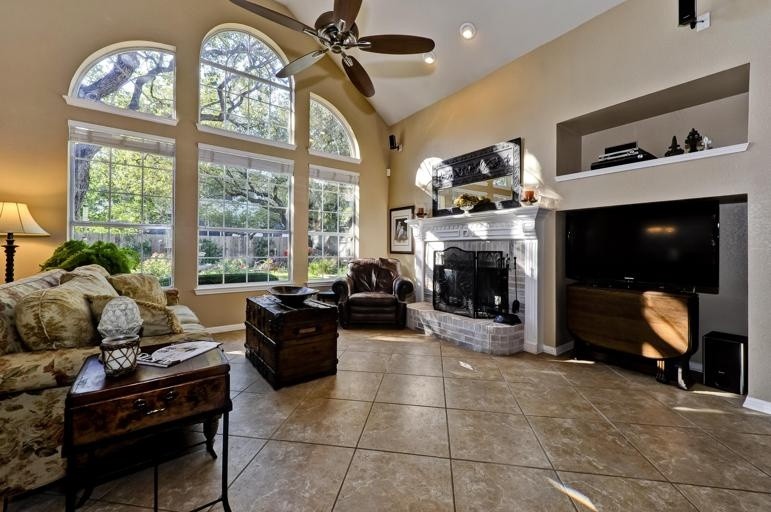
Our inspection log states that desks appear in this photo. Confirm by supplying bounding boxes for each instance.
[565,281,700,390]
[65,340,231,512]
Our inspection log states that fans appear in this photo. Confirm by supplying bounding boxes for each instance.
[229,0,435,98]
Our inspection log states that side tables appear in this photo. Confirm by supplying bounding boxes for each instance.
[319,292,340,325]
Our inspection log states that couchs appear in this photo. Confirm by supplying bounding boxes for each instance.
[1,267,234,490]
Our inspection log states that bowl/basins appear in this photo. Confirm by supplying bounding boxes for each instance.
[266,286,320,304]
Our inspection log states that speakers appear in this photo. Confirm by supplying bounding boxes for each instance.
[389,135,396,149]
[679,0,697,25]
[702,330,748,396]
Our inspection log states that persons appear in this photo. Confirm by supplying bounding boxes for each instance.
[398,226,405,241]
[397,221,402,234]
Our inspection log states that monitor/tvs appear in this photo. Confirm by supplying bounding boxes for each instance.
[565,199,719,294]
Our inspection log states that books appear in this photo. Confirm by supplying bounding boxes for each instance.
[136,336,224,368]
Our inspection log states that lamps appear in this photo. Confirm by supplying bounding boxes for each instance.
[459,23,476,40]
[100,297,143,373]
[422,51,436,66]
[0,203,50,283]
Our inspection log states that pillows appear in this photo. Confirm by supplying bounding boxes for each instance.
[106,273,166,307]
[84,296,183,341]
[59,263,111,282]
[16,276,119,350]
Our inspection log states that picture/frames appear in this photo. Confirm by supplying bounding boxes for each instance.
[389,207,415,254]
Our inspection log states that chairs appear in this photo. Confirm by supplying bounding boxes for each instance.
[333,260,413,329]
